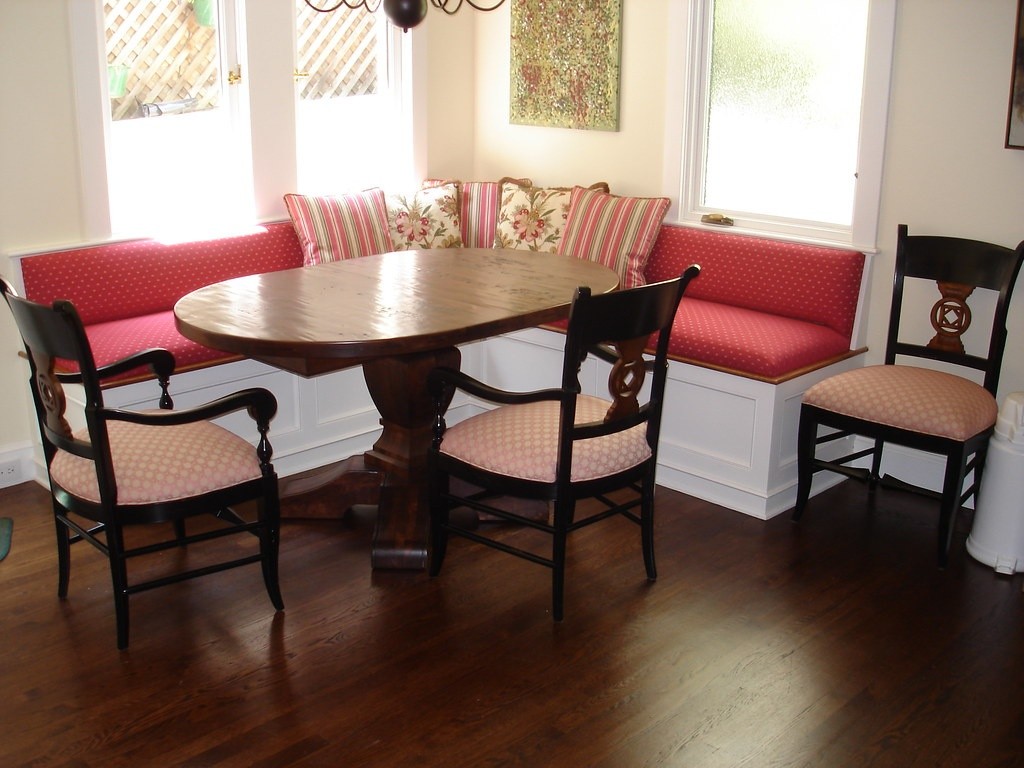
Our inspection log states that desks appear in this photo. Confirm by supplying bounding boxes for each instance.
[174,245,621,577]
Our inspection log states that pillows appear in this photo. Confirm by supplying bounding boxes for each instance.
[556,186,672,291]
[492,176,611,255]
[422,178,533,248]
[384,180,466,252]
[283,186,395,266]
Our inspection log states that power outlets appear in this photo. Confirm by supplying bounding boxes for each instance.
[0,458,23,485]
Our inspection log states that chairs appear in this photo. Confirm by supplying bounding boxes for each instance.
[793,224,1024,574]
[0,276,286,651]
[426,263,701,621]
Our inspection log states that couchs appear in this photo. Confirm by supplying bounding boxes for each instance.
[7,214,874,520]
